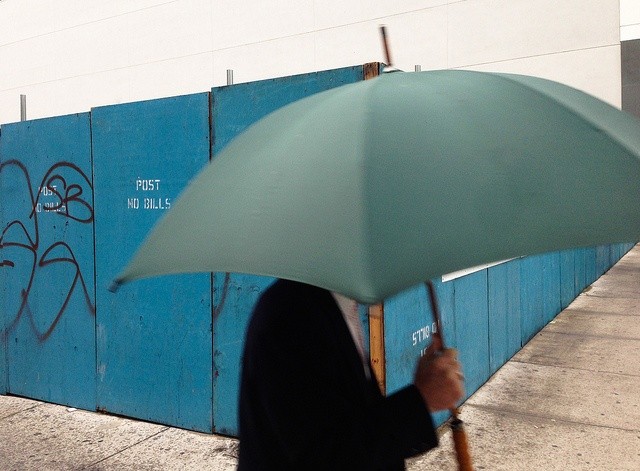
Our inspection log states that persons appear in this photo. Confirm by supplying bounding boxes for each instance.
[238,279,465,471]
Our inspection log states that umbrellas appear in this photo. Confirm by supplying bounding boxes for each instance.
[105,24,640,471]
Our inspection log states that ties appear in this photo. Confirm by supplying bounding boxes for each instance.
[345,301,373,378]
